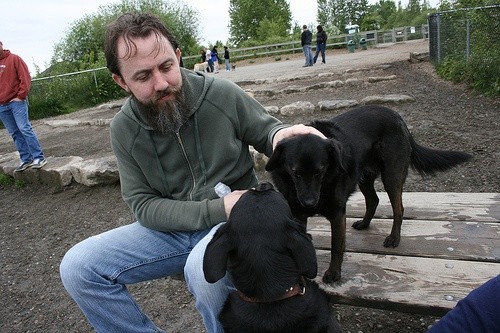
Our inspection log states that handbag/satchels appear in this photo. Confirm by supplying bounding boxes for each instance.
[217,56,223,64]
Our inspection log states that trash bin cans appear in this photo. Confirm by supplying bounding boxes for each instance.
[360,38,367,50]
[347,40,356,53]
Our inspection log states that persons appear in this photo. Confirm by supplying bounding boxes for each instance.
[313,26,327,64]
[200,46,220,74]
[427,274,500,333]
[59,12,328,333]
[0,41,48,172]
[222,46,230,72]
[300,25,314,67]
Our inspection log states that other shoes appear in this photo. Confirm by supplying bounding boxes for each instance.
[309,65,313,66]
[322,61,325,64]
[314,57,316,62]
[226,70,229,71]
[215,72,219,74]
[302,65,308,67]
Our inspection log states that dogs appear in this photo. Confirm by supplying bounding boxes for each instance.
[194,62,209,74]
[265,105,472,283]
[203,182,331,333]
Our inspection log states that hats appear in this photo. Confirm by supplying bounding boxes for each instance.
[214,46,218,48]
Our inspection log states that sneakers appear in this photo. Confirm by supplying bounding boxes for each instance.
[31,157,47,169]
[15,160,33,172]
